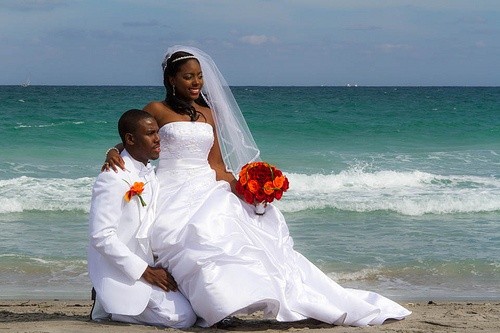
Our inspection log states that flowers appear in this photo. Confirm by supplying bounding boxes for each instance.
[122,178,146,207]
[236,162,289,214]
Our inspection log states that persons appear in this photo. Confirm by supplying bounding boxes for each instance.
[100,45,291,329]
[86,109,198,330]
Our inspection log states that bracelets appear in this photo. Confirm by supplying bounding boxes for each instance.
[106,147,120,155]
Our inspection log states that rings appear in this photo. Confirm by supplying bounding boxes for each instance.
[104,162,109,166]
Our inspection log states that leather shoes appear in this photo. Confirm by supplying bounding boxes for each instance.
[90,287,111,322]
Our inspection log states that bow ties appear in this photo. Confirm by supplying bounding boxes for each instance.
[138,165,156,178]
